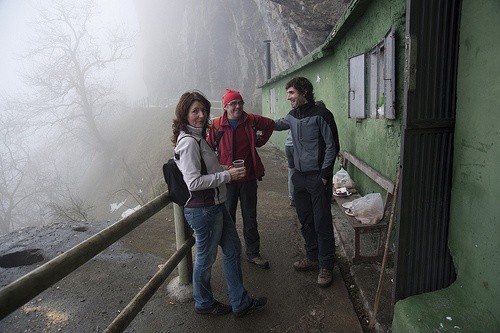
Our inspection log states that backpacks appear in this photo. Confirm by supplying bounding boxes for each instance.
[162,135,208,208]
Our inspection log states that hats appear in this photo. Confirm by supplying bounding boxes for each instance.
[222,89,243,106]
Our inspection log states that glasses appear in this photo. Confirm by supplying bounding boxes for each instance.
[227,100,244,106]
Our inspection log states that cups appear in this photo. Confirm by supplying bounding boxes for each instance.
[233,160,244,175]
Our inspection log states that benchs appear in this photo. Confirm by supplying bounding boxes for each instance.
[331,151,397,266]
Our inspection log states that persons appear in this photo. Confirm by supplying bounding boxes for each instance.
[171,89,268,317]
[272,77,340,287]
[207,90,276,270]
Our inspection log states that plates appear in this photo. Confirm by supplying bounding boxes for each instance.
[345,209,354,216]
[333,191,352,197]
[342,202,351,208]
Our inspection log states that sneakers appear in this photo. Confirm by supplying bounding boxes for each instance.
[318,269,333,288]
[196,301,232,315]
[293,258,319,272]
[246,256,269,270]
[234,296,268,318]
